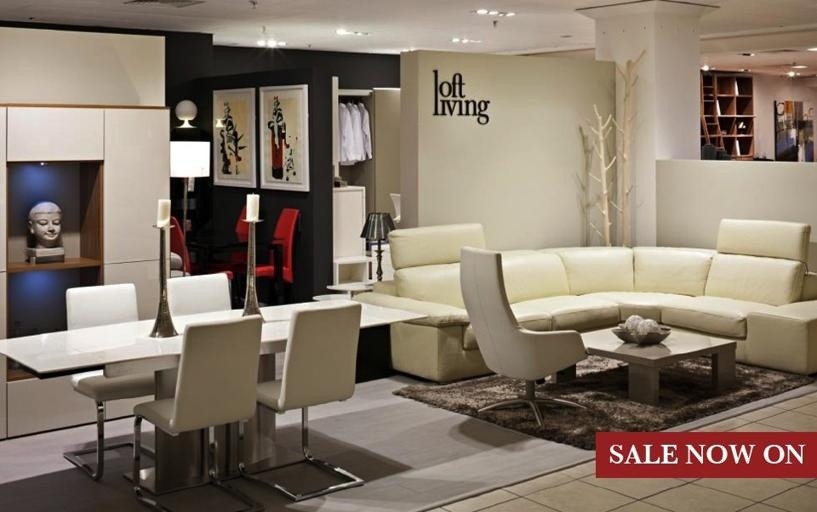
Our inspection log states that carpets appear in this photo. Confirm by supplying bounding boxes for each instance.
[392,355,815,450]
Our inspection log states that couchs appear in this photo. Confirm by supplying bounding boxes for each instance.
[351,219,817,385]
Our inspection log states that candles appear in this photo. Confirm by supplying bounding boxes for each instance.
[157,199,171,228]
[246,193,260,221]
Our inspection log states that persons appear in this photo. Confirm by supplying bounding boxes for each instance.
[775,102,785,117]
[806,107,814,119]
[27,198,62,250]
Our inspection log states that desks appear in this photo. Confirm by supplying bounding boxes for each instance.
[0,300,428,497]
[326,280,378,299]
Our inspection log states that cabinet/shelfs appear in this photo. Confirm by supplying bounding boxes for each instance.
[332,77,401,283]
[700,70,755,160]
[7,161,105,380]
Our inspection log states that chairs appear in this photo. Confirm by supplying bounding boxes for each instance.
[170,205,301,307]
[134,314,266,512]
[460,247,589,428]
[63,283,155,480]
[237,304,366,502]
[166,273,232,317]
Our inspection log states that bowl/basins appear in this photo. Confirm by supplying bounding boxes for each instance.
[630,332,669,344]
[610,328,671,344]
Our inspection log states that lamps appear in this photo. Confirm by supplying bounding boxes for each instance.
[170,100,211,277]
[359,213,396,282]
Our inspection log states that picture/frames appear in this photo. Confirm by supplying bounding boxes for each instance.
[259,84,311,192]
[212,87,257,188]
